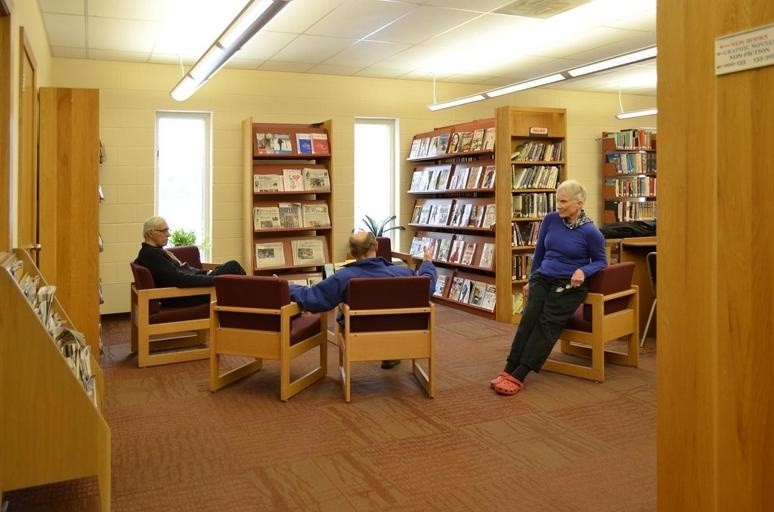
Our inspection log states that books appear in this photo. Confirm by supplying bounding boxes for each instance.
[5,260,100,409]
[249,131,333,292]
[409,127,496,313]
[512,141,566,318]
[606,128,656,222]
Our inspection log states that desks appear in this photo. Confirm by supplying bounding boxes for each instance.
[621,237,655,338]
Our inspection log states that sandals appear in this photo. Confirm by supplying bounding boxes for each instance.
[495,375,523,395]
[489,371,509,389]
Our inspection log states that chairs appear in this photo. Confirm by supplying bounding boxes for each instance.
[523,252,655,385]
[208,237,436,400]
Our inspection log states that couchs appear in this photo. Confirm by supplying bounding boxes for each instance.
[126,244,225,366]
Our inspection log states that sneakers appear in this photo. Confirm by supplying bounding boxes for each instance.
[380,360,401,369]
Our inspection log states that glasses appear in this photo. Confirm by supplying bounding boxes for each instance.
[154,228,169,232]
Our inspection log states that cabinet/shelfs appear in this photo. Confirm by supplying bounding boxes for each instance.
[600,127,657,229]
[240,115,333,281]
[406,105,568,326]
[36,86,100,373]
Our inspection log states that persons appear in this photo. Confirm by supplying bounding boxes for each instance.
[134,216,248,309]
[489,180,608,396]
[273,230,438,371]
